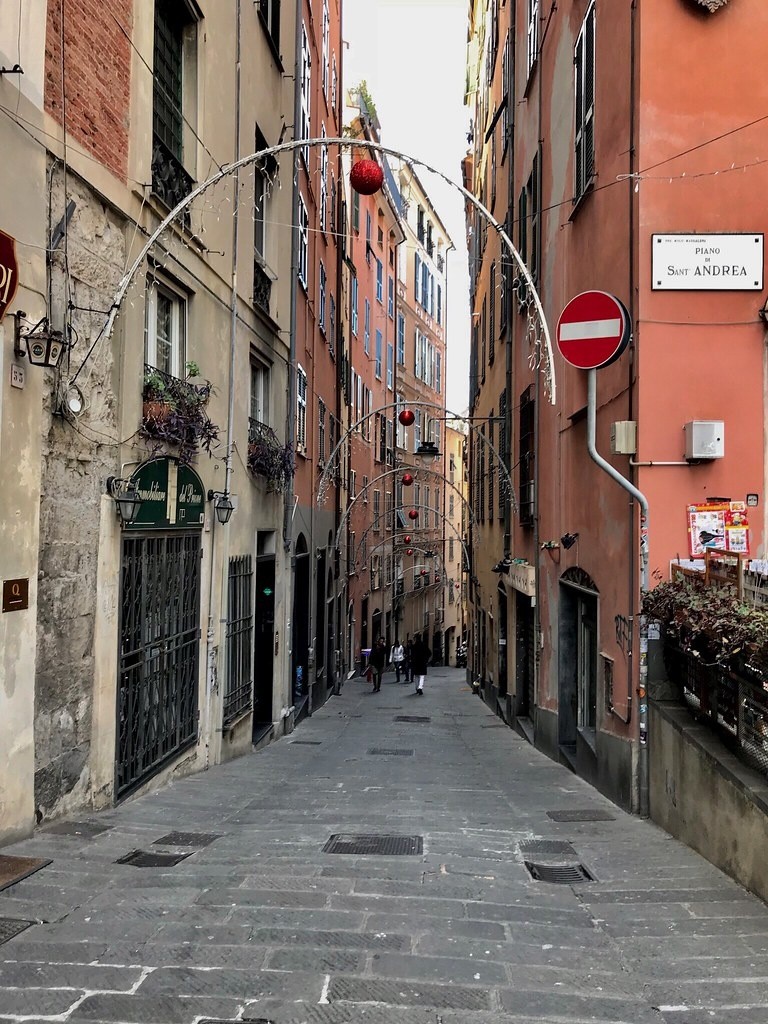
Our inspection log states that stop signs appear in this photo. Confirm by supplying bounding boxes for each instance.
[555,286,628,370]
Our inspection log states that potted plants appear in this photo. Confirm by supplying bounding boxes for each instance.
[143,370,173,420]
[248,436,258,453]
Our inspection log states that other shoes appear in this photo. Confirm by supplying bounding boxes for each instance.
[417,689,423,695]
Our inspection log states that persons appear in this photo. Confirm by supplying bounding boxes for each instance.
[389,640,415,683]
[456,642,467,667]
[411,632,431,695]
[368,636,390,693]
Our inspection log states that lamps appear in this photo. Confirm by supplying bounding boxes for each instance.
[14,310,68,367]
[208,489,235,525]
[412,442,443,465]
[107,476,143,522]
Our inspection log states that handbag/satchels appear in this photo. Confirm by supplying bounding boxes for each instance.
[367,669,371,682]
